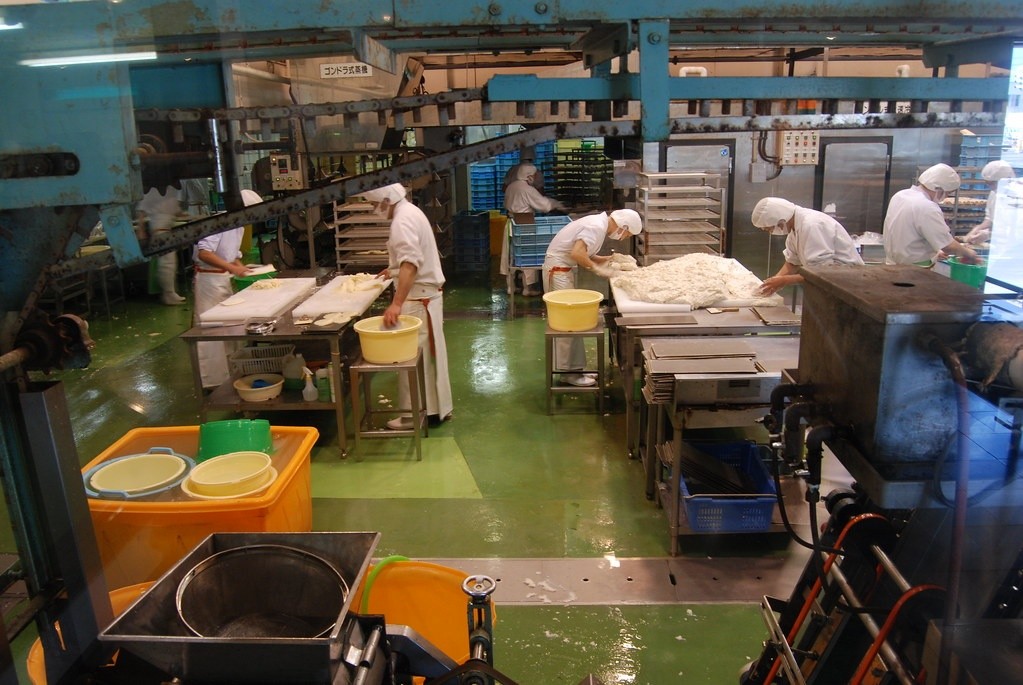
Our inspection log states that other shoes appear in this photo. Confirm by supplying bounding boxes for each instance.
[559,374,598,386]
[507,288,521,294]
[387,417,421,430]
[523,289,542,296]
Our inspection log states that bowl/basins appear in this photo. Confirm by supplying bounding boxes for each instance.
[353,314,422,364]
[82,419,278,500]
[79,245,109,256]
[543,288,604,331]
[233,373,284,401]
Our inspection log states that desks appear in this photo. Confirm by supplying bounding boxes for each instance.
[545,318,605,415]
[349,350,430,459]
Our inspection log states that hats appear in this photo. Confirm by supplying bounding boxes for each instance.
[919,163,960,191]
[752,197,794,227]
[517,163,537,179]
[610,209,642,234]
[363,183,407,204]
[982,160,1016,181]
[520,146,535,160]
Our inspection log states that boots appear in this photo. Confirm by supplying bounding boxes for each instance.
[160,274,186,305]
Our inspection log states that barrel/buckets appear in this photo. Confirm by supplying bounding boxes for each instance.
[234,271,278,289]
[948,257,988,289]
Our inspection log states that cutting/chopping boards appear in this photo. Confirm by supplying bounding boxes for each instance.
[709,258,783,307]
[200,278,316,321]
[292,274,393,317]
[609,270,691,313]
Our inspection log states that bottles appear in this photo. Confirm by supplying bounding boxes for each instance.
[282,352,306,390]
[316,364,329,401]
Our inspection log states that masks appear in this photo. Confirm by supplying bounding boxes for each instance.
[933,189,945,203]
[608,226,625,240]
[528,176,535,185]
[374,200,390,221]
[770,221,788,235]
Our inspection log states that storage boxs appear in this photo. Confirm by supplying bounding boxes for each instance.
[449,143,573,267]
[77,426,320,590]
[958,134,1002,190]
[665,440,780,534]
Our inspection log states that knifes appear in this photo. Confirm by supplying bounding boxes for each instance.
[198,320,245,327]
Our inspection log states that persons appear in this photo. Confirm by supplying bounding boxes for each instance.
[751,196,860,297]
[964,161,1016,245]
[541,209,642,386]
[502,147,545,197]
[192,190,263,403]
[136,168,191,306]
[883,163,977,270]
[500,164,554,296]
[177,178,211,217]
[365,183,454,430]
